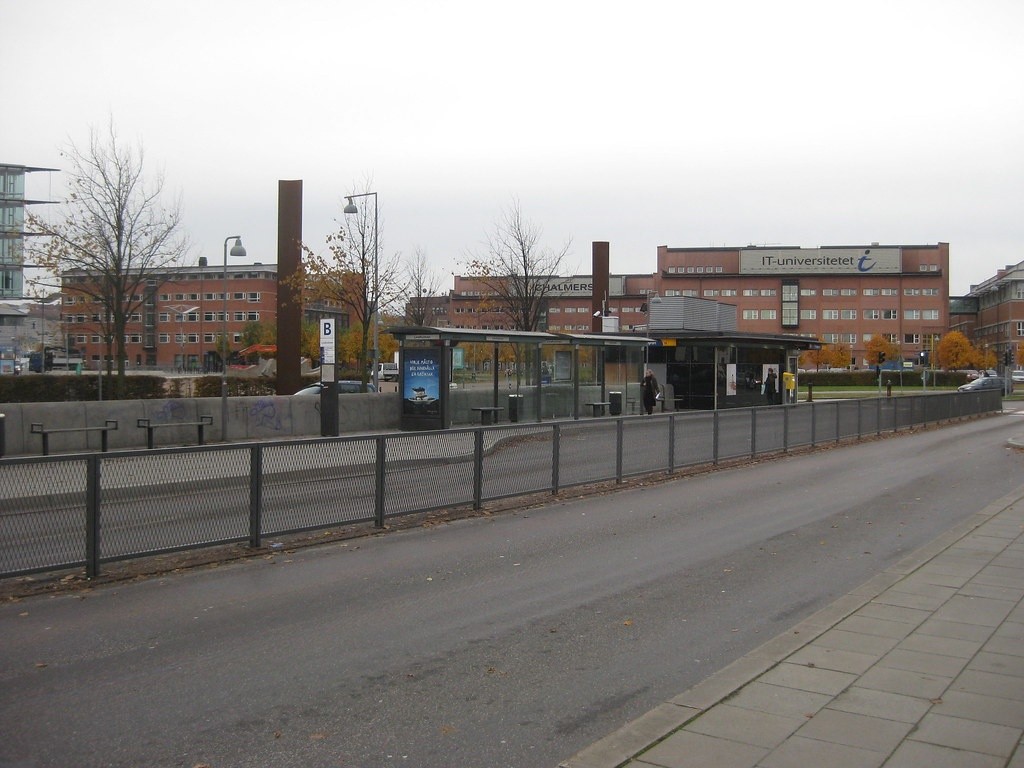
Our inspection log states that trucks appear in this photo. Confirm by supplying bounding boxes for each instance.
[371,362,399,382]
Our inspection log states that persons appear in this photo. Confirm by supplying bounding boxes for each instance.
[979,369,990,378]
[764,368,778,405]
[640,369,661,416]
[967,373,974,384]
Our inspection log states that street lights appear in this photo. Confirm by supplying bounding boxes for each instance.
[642,290,662,371]
[341,192,381,394]
[163,305,201,373]
[932,334,941,389]
[22,292,70,374]
[221,234,247,444]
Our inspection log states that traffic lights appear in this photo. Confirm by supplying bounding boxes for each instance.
[1002,351,1008,366]
[1008,347,1015,365]
[878,351,886,365]
[920,352,925,357]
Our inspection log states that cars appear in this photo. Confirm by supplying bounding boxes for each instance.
[1011,370,1024,384]
[957,369,980,380]
[978,369,997,378]
[957,376,1014,396]
[293,378,381,396]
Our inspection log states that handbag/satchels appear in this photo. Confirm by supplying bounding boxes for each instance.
[761,384,766,395]
